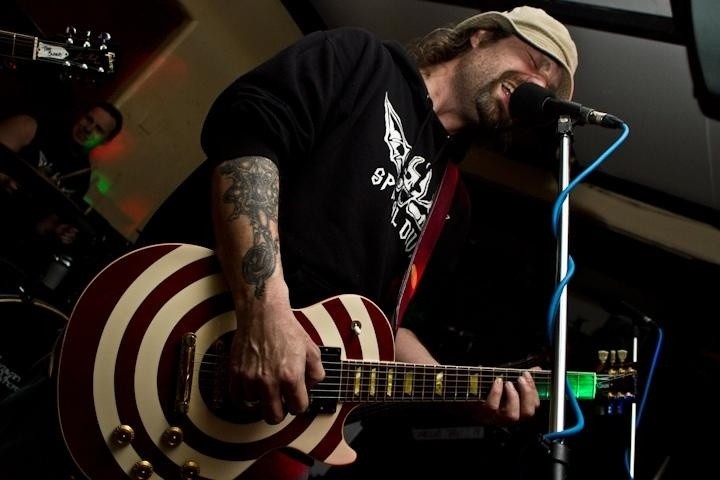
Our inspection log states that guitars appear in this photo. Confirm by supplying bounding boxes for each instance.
[53,239,640,479]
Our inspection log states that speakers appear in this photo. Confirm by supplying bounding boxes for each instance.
[72,200,134,285]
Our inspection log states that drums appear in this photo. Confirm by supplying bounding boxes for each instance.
[0,282,73,480]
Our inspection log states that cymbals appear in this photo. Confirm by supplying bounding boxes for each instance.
[1,142,69,205]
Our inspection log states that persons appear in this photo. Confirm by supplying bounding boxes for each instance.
[132,6,579,479]
[0,99,122,246]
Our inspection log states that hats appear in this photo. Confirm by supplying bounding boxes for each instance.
[455,6,578,102]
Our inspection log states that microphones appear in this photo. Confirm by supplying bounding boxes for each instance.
[599,287,652,324]
[508,82,624,129]
